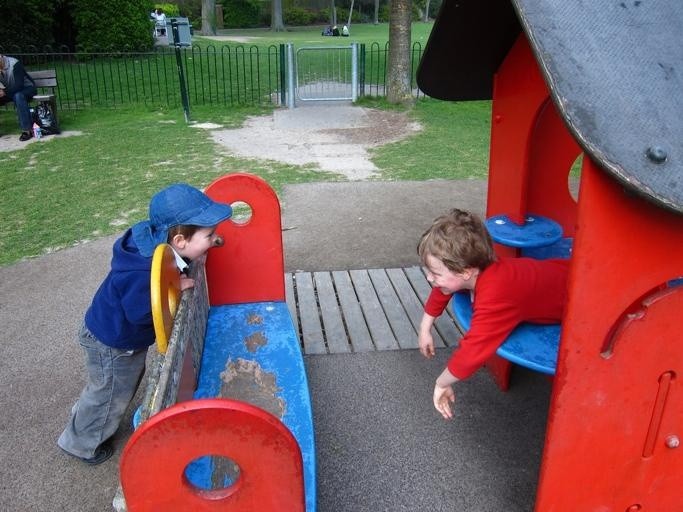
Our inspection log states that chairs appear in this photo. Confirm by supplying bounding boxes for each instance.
[154,19,168,37]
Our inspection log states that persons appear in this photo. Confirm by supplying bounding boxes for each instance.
[55,181,235,466]
[0,54,37,142]
[149,9,159,32]
[155,9,166,37]
[413,208,573,421]
[321,26,350,38]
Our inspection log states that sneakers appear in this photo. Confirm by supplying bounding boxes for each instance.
[58,444,112,464]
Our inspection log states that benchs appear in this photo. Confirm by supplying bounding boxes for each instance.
[0,70,60,134]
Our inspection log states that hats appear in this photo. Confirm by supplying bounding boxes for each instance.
[132,183,233,258]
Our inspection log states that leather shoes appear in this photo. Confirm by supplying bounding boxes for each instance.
[20,131,33,141]
